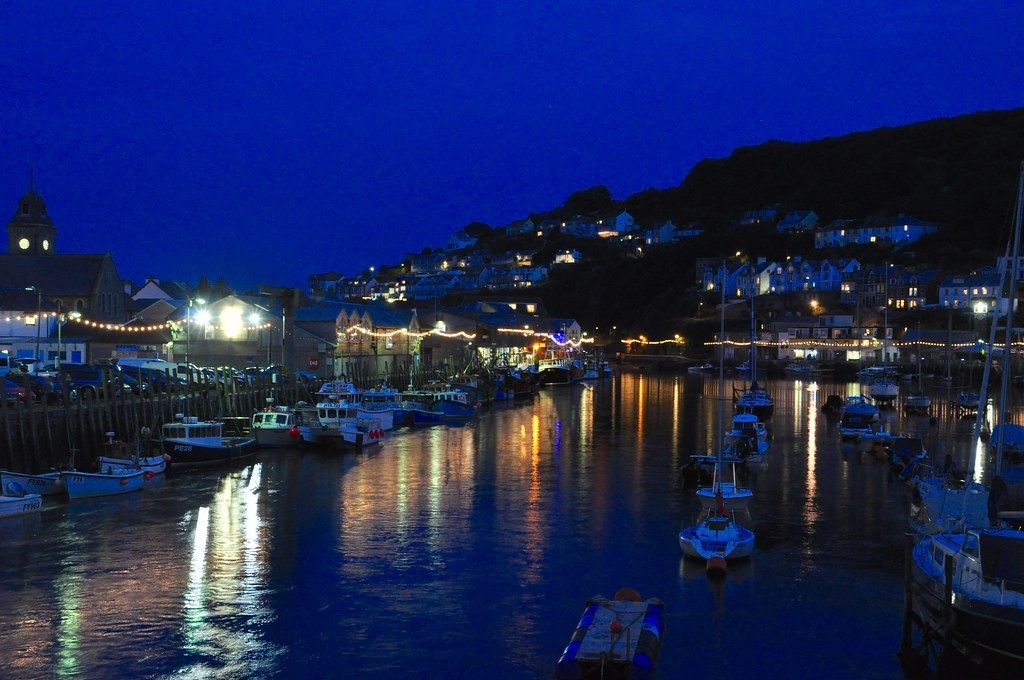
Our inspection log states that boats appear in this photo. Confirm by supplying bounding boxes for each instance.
[159,411,259,468]
[0,449,166,517]
[252,353,613,447]
[678,258,774,582]
[556,585,674,680]
[784,184,1023,680]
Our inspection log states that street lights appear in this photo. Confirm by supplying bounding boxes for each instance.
[185,295,207,397]
